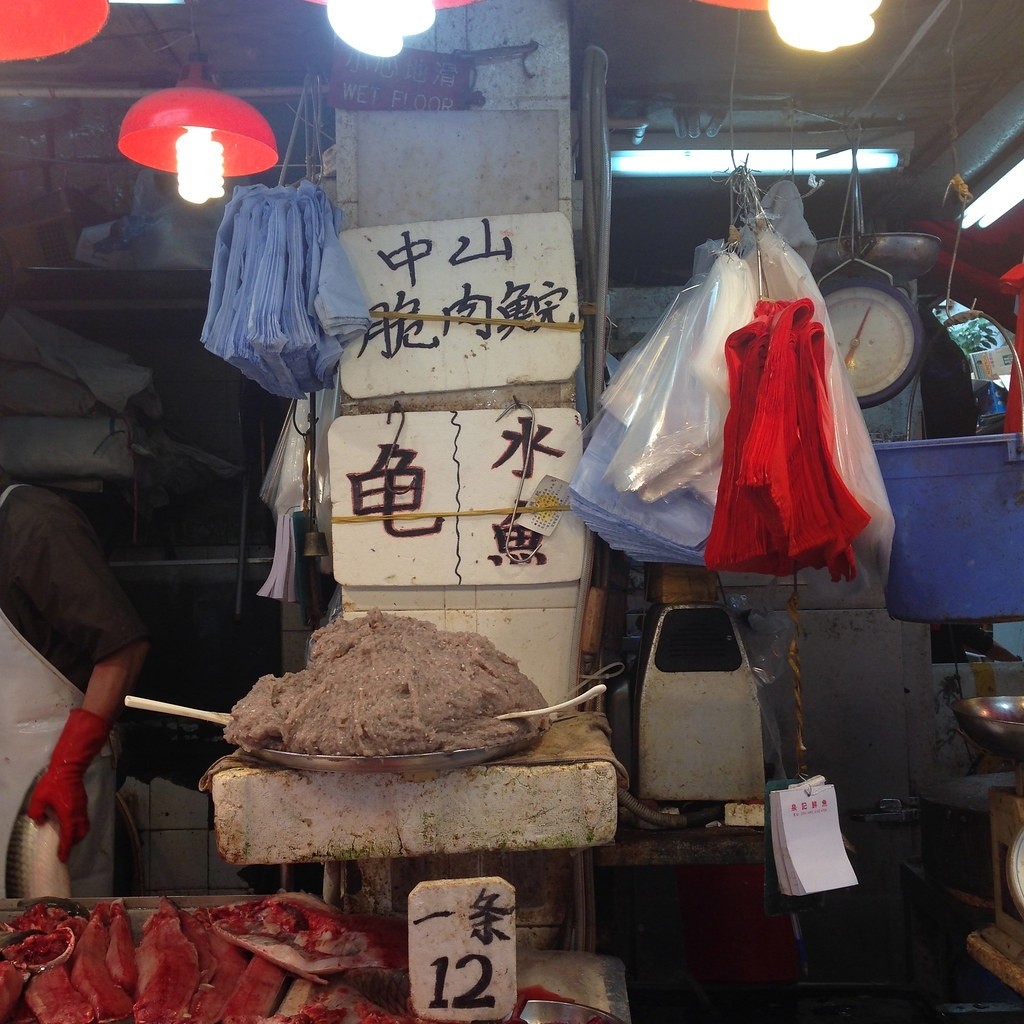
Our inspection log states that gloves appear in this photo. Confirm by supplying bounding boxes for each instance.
[28,707,111,862]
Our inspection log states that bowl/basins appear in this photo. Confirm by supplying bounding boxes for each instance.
[507,1000,626,1024]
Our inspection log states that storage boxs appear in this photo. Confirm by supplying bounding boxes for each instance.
[869,431,1023,625]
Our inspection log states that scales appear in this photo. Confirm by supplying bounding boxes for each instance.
[809,234,943,441]
[950,695,1024,994]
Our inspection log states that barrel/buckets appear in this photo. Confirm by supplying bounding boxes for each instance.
[873,309,1023,622]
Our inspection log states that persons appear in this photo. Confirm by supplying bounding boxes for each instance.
[1,468,150,899]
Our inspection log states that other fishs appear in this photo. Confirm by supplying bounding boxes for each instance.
[0,890,412,1024]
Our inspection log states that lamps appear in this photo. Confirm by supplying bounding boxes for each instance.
[608,129,912,183]
[952,144,1024,228]
